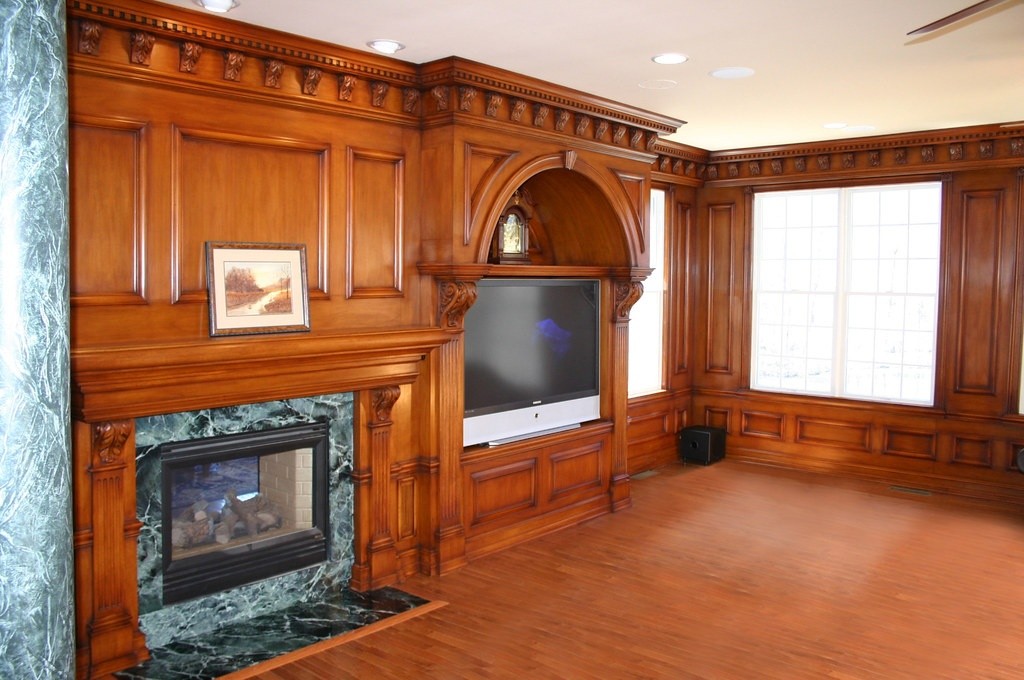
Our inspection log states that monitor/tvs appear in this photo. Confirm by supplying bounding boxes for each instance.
[463,279,602,448]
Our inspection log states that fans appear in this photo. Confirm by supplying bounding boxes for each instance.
[907,0,1006,36]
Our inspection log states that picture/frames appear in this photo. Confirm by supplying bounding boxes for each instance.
[204,241,311,336]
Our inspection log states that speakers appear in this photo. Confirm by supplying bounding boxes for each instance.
[680,425,725,465]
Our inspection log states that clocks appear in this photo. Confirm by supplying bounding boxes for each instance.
[489,186,538,264]
